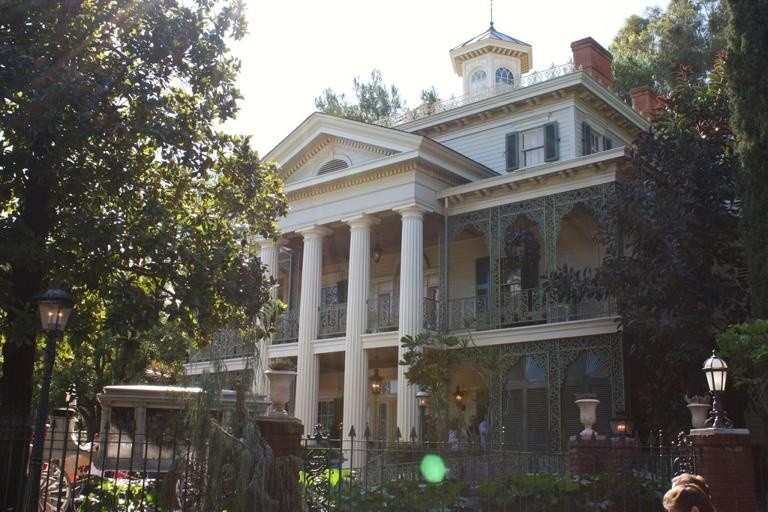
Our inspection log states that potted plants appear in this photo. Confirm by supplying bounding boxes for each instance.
[262,356,298,417]
[573,392,600,433]
[684,393,711,429]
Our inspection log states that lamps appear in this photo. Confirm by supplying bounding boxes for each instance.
[701,349,731,428]
[368,350,385,395]
[609,410,636,437]
[373,232,384,263]
[452,384,465,411]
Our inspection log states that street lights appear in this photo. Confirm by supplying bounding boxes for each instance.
[21,287,74,511]
[415,388,431,440]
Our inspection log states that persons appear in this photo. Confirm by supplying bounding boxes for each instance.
[448,416,466,451]
[671,475,712,500]
[662,484,716,512]
[479,413,490,447]
